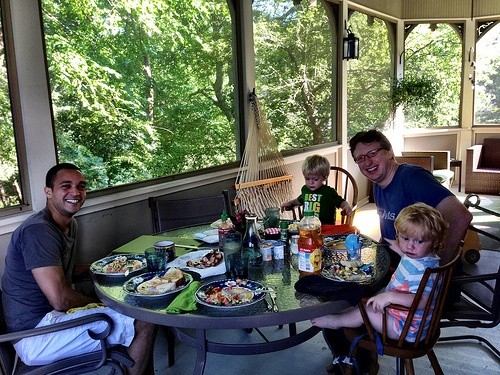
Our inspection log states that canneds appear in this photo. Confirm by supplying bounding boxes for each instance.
[264,228,281,242]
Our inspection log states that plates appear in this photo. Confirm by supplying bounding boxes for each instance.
[322,261,376,283]
[210,217,231,229]
[322,234,363,252]
[89,228,267,309]
[259,220,300,250]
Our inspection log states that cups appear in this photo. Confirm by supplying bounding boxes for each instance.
[274,242,286,260]
[227,253,249,279]
[259,244,274,262]
[222,232,242,275]
[242,247,263,280]
[153,240,176,264]
[144,247,166,271]
[264,207,280,228]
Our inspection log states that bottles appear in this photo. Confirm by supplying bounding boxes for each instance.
[280,220,289,241]
[297,209,322,278]
[219,190,264,281]
[289,235,300,254]
[345,233,363,266]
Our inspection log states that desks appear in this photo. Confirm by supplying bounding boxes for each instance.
[450,160,462,192]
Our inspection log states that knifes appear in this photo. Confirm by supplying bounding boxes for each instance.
[268,287,278,313]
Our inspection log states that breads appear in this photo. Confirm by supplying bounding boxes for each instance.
[162,266,184,284]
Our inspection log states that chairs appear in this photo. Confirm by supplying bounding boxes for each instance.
[283,166,500,375]
[147,190,232,233]
[0,284,135,375]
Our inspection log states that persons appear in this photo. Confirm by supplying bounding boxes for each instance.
[281,155,352,224]
[311,203,449,343]
[1,163,158,375]
[322,130,473,375]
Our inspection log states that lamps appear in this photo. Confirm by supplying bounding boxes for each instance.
[342,30,359,60]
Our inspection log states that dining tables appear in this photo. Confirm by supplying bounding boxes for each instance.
[95,222,391,375]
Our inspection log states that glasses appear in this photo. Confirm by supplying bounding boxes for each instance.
[354,148,384,163]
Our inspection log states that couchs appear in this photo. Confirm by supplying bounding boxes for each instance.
[465,138,500,196]
[382,131,454,190]
